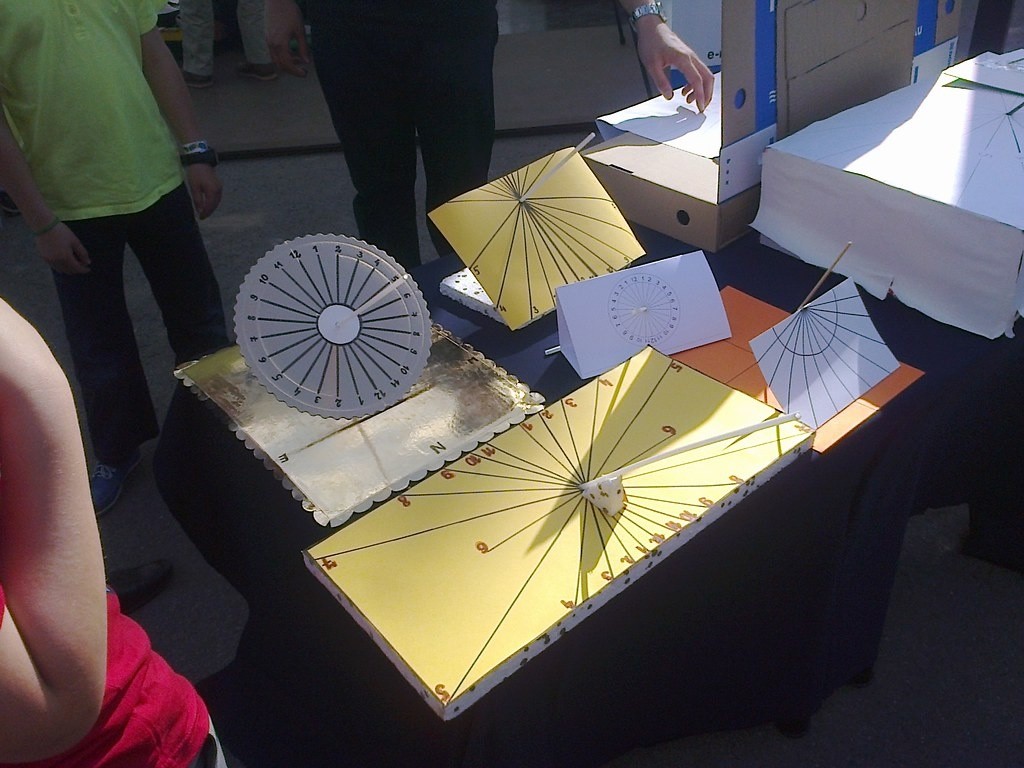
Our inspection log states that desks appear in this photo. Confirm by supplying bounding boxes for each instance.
[151,223,1024,768]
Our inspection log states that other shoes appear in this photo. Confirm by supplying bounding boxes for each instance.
[0,190,20,213]
[235,63,278,81]
[181,69,213,88]
[106,560,174,613]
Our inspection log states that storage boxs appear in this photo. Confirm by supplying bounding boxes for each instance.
[581,0,978,252]
[751,46,1024,348]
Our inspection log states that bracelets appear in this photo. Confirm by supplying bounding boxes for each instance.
[183,140,208,154]
[33,217,59,236]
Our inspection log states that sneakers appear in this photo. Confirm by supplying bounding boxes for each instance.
[90,444,143,516]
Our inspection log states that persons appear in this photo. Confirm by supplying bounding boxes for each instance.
[0,0,234,514]
[179,0,279,88]
[260,0,714,279]
[0,297,227,768]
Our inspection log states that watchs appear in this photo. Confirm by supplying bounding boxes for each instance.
[629,2,668,31]
[180,149,218,168]
